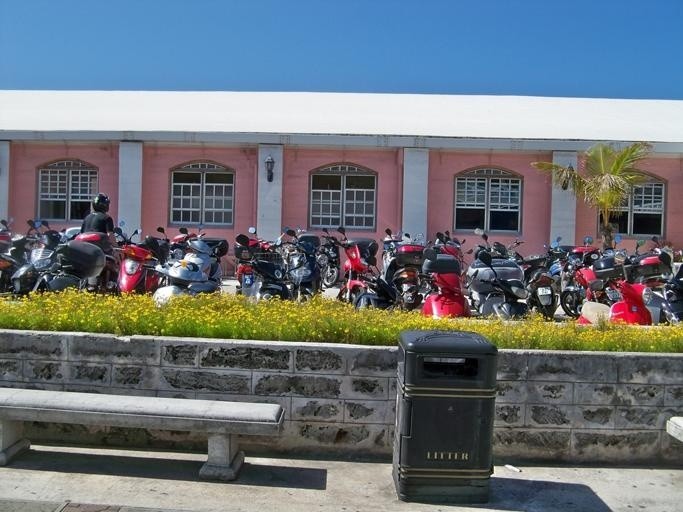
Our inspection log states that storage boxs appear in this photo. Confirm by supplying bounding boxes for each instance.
[666,416,683,442]
[0,388,285,481]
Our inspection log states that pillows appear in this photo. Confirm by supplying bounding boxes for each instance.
[92,193,110,212]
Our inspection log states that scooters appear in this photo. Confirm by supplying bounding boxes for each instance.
[0,215,683,328]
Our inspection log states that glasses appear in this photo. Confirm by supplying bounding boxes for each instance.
[264,154,274,181]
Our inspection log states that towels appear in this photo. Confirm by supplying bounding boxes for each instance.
[391,330,499,504]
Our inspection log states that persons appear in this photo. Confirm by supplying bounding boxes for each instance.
[81,193,116,246]
